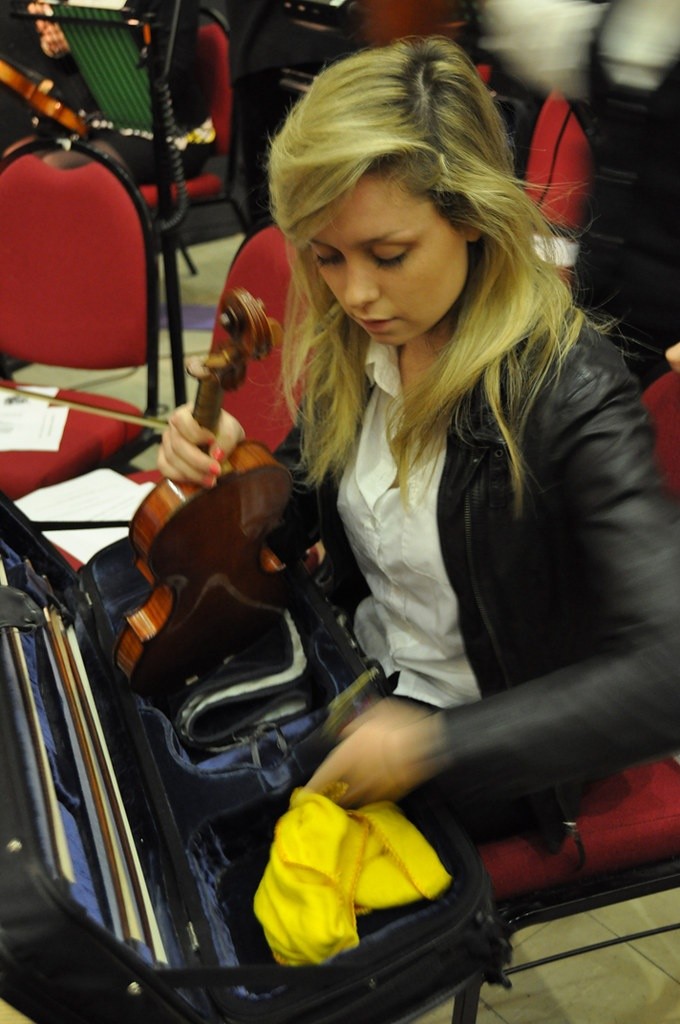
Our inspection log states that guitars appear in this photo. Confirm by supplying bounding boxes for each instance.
[0,49,92,145]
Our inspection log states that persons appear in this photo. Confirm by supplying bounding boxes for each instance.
[0,0,680,845]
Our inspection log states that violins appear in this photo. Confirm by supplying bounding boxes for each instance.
[113,283,296,703]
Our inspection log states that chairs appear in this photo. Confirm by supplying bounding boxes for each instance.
[0,0,680,1024]
